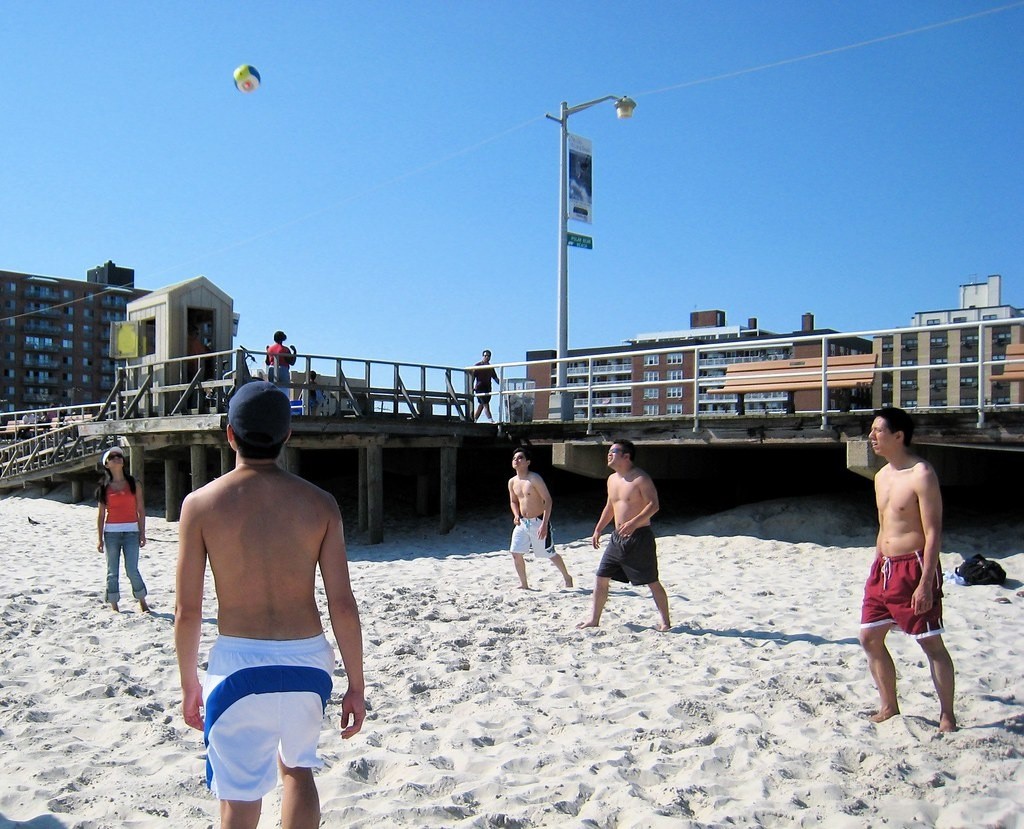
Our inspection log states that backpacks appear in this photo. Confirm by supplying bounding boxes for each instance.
[955,554,1007,586]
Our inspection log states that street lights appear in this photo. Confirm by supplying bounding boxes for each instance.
[543,93,638,423]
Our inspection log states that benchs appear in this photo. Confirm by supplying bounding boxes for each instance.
[989,344,1024,382]
[0,414,93,441]
[706,354,878,415]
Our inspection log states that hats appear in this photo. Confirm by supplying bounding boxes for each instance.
[103,447,123,465]
[228,379,291,446]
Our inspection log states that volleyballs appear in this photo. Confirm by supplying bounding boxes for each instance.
[235,64,259,93]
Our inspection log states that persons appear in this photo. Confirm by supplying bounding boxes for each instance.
[0,409,8,443]
[858,407,957,732]
[508,447,573,589]
[470,350,499,425]
[94,445,152,615]
[297,371,317,417]
[8,403,66,444]
[579,439,672,631]
[265,331,297,400]
[172,381,365,829]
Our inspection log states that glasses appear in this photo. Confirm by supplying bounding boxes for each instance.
[105,454,123,464]
[609,448,625,453]
